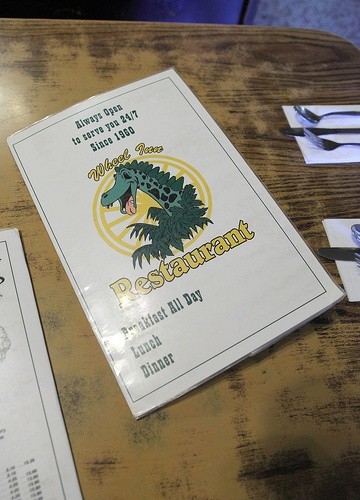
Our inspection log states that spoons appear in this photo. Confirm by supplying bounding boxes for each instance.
[294,104,360,124]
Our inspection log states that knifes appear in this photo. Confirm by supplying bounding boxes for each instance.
[278,127,360,138]
[318,247,360,261]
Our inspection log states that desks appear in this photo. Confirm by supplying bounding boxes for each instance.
[1,18,360,500]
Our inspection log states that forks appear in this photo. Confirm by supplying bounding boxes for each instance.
[302,127,360,151]
